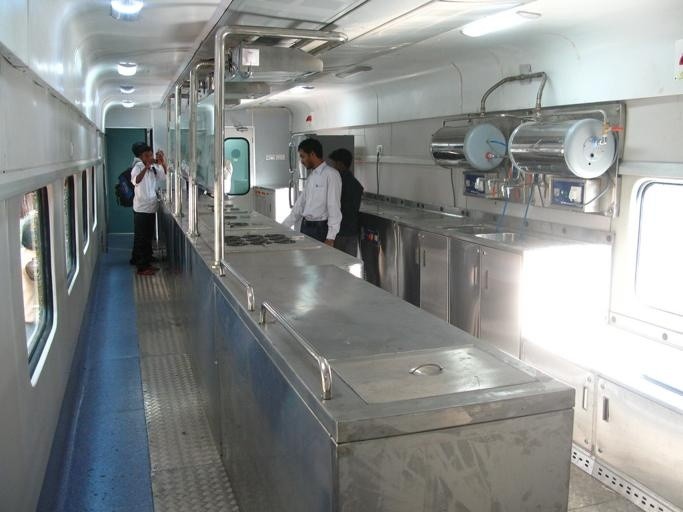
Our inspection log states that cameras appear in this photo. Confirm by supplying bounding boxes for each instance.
[152,159,159,163]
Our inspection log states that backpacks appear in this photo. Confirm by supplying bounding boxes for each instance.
[114,167,136,208]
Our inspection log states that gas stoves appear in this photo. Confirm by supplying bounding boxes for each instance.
[206,202,317,252]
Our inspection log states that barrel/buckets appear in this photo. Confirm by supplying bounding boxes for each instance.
[429,125,506,172]
[507,120,615,180]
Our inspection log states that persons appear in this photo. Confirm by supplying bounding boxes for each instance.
[129,146,167,276]
[328,148,364,257]
[206,144,235,200]
[280,138,343,246]
[128,141,164,266]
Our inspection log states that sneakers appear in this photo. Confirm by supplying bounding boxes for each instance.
[129,248,160,276]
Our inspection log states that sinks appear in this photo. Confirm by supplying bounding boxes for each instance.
[476,232,548,249]
[443,225,511,235]
